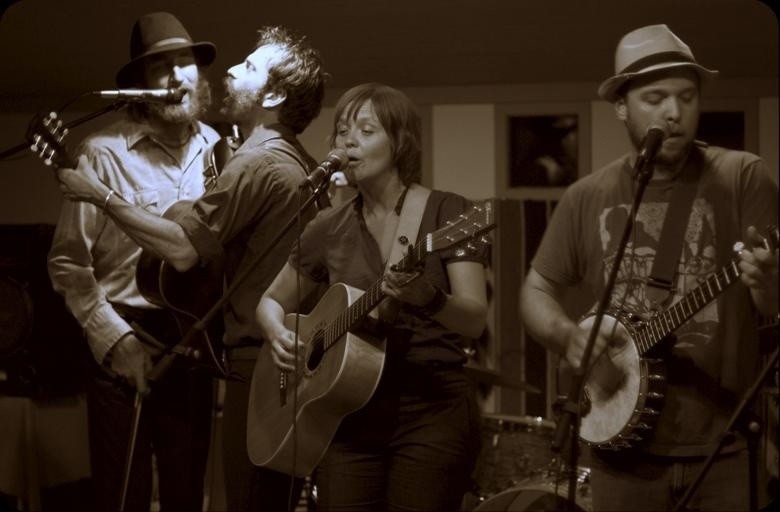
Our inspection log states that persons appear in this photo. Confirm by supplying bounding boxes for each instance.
[46,10,228,512]
[254,83,488,510]
[57,27,328,512]
[520,24,780,510]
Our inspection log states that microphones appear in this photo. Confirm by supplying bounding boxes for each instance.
[630,117,672,183]
[91,86,183,104]
[298,148,349,195]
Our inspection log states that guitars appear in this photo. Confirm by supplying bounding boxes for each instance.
[28,112,252,381]
[247,199,495,479]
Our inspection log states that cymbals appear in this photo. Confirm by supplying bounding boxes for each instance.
[462,362,542,395]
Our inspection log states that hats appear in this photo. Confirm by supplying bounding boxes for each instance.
[114,12,219,89]
[600,25,718,104]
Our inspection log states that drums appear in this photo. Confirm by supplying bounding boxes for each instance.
[470,464,594,512]
[468,413,557,497]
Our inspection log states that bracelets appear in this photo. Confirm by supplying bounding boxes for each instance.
[417,284,447,318]
[102,188,116,211]
